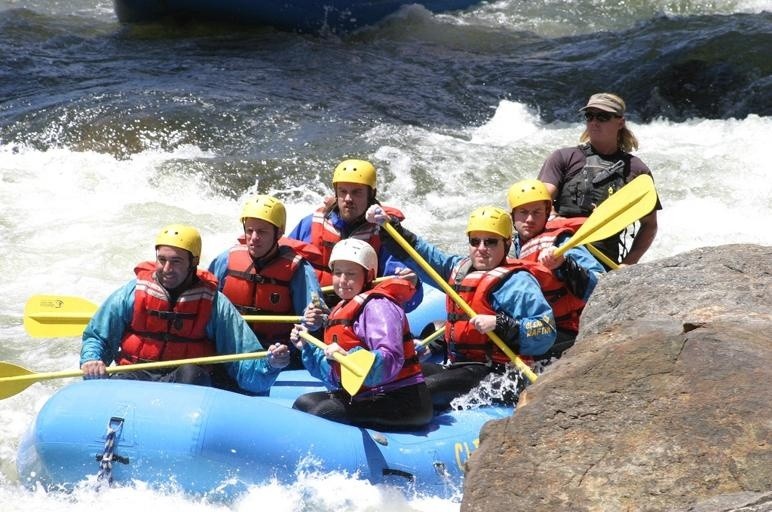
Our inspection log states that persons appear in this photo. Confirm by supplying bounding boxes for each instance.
[363,201,557,408]
[289,156,427,314]
[535,92,663,271]
[79,223,290,394]
[502,177,606,356]
[291,237,433,430]
[207,192,329,360]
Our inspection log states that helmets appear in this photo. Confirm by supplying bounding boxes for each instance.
[239,194,285,234]
[508,179,552,213]
[465,207,512,245]
[327,239,377,279]
[155,223,202,264]
[332,158,376,192]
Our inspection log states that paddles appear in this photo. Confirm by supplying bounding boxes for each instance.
[413,174,658,353]
[0,342,281,400]
[297,330,375,397]
[23,292,320,340]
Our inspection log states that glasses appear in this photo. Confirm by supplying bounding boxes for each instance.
[584,112,611,122]
[469,237,501,247]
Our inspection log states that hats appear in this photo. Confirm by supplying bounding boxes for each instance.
[578,93,624,117]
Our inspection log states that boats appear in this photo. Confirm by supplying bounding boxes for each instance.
[112,0,491,38]
[33,258,516,512]
